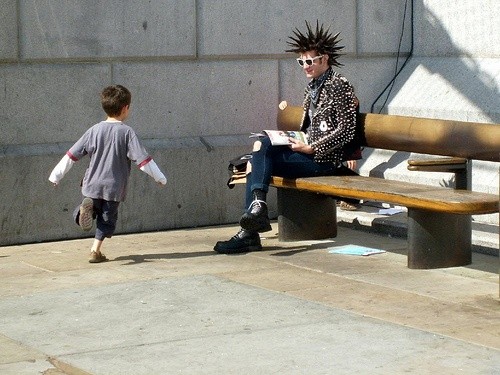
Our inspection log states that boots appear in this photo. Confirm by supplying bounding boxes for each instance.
[239,190,272,233]
[213,228,262,253]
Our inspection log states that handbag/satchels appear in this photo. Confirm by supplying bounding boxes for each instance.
[226,153,253,189]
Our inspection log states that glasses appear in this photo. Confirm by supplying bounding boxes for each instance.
[297,55,324,67]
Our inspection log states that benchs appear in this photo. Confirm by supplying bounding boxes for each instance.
[271,106,500,270]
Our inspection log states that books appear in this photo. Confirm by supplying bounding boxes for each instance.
[249,129,310,147]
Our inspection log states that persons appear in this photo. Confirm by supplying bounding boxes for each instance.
[48,83,168,263]
[213,19,356,253]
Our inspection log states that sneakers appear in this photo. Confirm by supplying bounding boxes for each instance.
[88,250,106,263]
[79,197,93,231]
